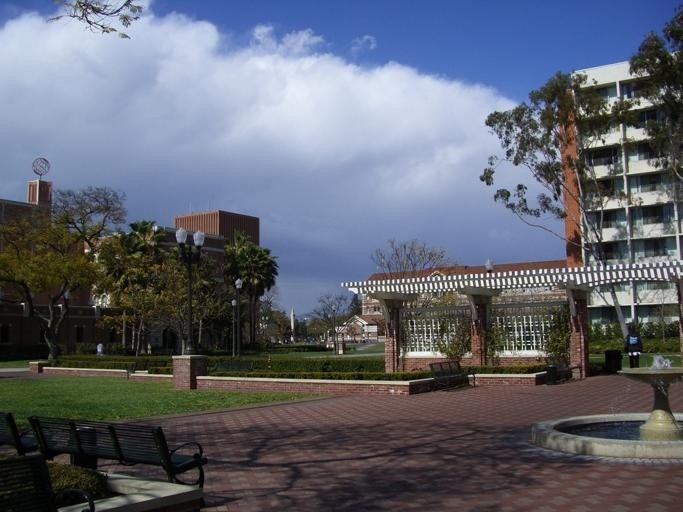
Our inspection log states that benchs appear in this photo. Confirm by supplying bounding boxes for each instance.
[429,360,476,393]
[1,409,210,512]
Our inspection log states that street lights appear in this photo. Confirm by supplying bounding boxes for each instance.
[234,279,242,358]
[484,257,494,289]
[331,304,335,355]
[231,299,236,358]
[261,315,268,344]
[175,227,204,355]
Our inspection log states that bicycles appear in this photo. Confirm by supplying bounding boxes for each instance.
[349,339,378,343]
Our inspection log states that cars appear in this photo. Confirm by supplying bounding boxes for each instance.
[265,334,335,345]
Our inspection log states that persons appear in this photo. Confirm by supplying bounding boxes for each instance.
[624,324,643,368]
[96,341,104,355]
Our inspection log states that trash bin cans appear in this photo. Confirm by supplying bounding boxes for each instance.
[605,350,622,374]
[70,427,97,471]
